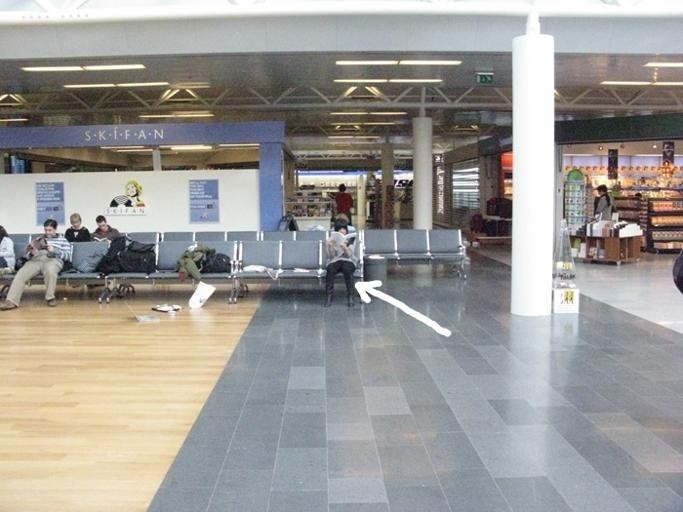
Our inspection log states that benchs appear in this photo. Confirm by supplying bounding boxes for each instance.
[362,229,466,280]
[106,241,237,303]
[64,231,159,242]
[234,240,364,303]
[161,231,259,241]
[0,234,110,303]
[260,230,361,240]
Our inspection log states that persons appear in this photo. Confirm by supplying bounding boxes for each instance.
[594,184,617,221]
[0,224,16,297]
[0,219,71,310]
[335,184,353,226]
[65,212,90,242]
[124,180,145,207]
[324,218,358,307]
[90,215,121,242]
[331,212,356,230]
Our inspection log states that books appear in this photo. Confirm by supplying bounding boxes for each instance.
[22,236,48,260]
[330,231,357,253]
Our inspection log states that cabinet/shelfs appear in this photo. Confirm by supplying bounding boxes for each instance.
[614,197,643,221]
[648,198,683,253]
[568,235,644,265]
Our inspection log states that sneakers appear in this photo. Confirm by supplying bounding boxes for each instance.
[46,298,56,306]
[323,295,333,307]
[346,294,355,307]
[0,302,17,310]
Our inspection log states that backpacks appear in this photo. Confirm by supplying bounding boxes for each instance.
[121,241,156,274]
[200,252,231,274]
[93,236,131,276]
[75,237,111,274]
